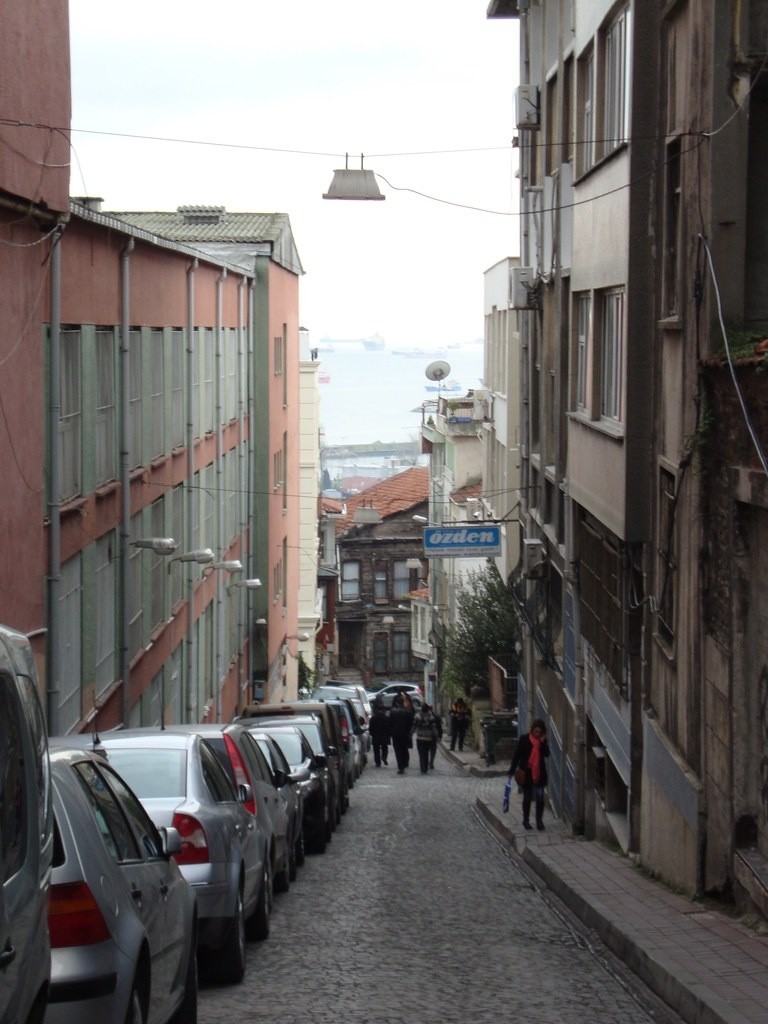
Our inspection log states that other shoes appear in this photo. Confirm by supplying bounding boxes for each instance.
[536,820,545,830]
[523,819,533,830]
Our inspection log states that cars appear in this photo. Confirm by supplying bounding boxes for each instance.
[44,747,201,1024]
[45,727,271,983]
[111,722,291,909]
[366,682,424,710]
[225,682,374,880]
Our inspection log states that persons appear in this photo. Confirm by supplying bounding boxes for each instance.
[507,719,550,831]
[369,689,443,774]
[448,698,469,751]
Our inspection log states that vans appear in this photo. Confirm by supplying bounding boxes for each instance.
[0,624,56,1024]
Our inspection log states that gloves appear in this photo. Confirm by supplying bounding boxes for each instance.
[507,770,512,777]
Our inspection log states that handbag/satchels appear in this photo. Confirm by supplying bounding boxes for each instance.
[502,776,511,812]
[515,770,526,788]
